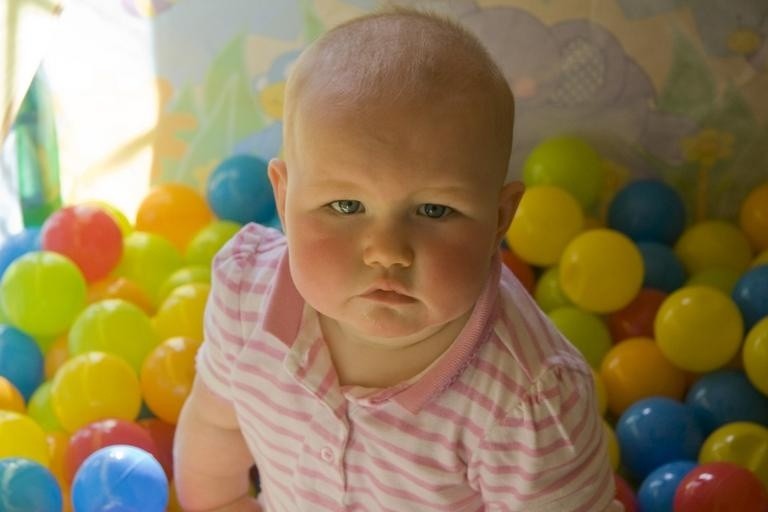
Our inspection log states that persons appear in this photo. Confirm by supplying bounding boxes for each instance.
[171,10,624,511]
